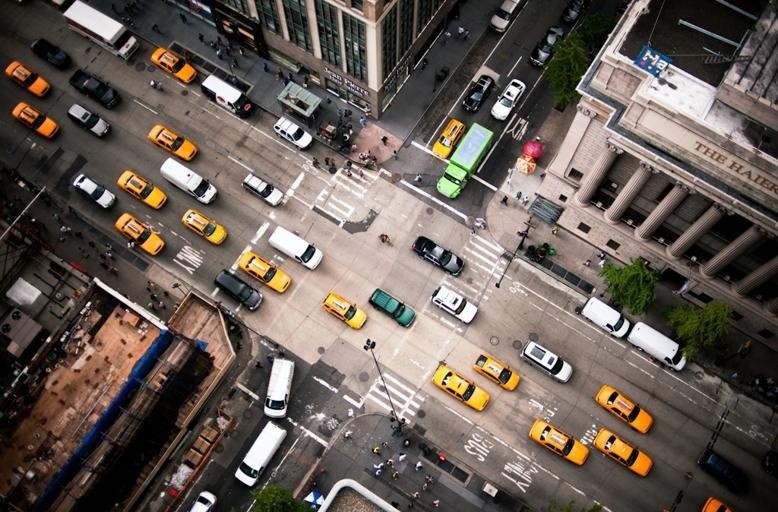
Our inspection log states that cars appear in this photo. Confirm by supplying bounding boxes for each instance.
[696,449,751,494]
[326,237,691,482]
[1,1,324,316]
[190,490,220,511]
[699,496,733,512]
[432,0,588,202]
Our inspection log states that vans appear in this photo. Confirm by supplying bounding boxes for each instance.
[231,422,288,488]
[264,356,296,420]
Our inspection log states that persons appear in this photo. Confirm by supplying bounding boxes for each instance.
[1,180,172,315]
[379,232,396,248]
[469,191,606,270]
[344,406,442,511]
[111,1,472,188]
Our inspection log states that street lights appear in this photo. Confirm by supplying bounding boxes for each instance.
[327,97,344,132]
